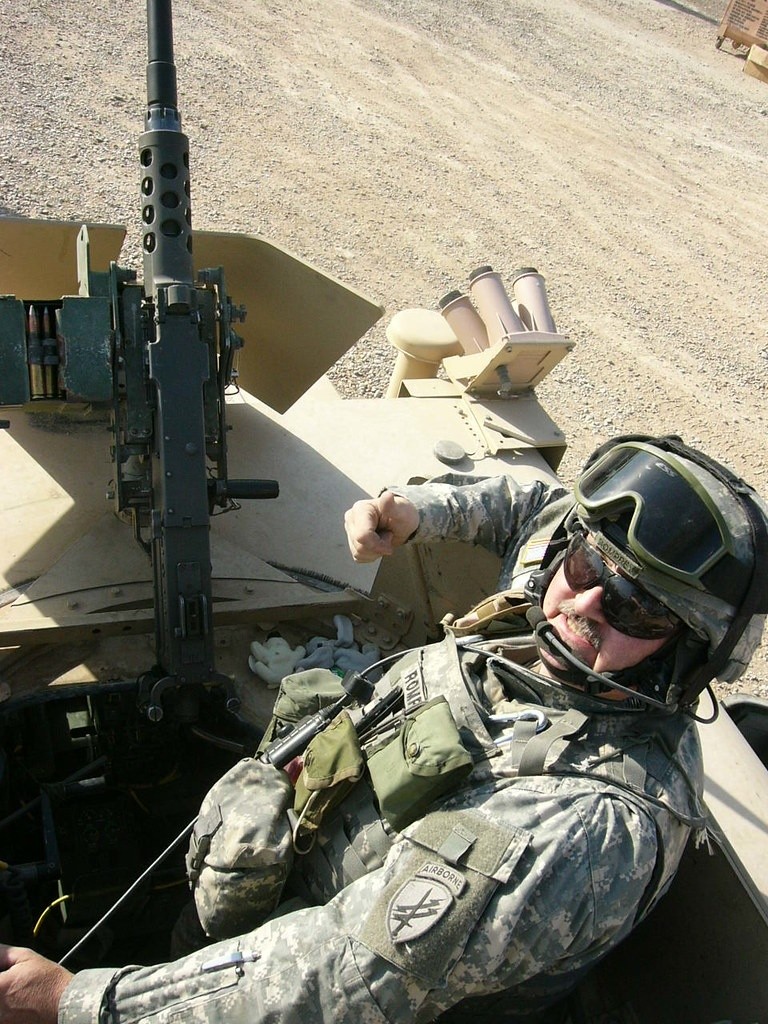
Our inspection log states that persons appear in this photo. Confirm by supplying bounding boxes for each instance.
[0,435,768,1024]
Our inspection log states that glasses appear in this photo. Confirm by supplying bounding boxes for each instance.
[563,532,679,639]
[575,442,728,591]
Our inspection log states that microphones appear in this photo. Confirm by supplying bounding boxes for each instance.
[527,605,679,714]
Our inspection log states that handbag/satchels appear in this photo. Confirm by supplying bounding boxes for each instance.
[252,667,362,840]
[185,758,298,941]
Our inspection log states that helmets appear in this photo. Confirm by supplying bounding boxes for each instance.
[575,435,768,685]
